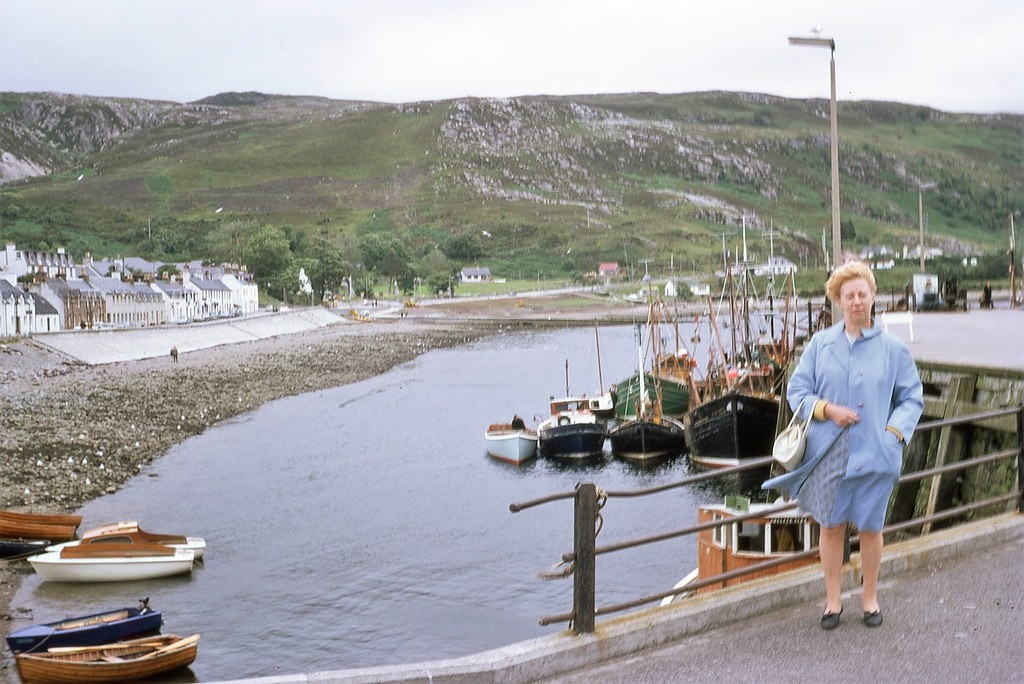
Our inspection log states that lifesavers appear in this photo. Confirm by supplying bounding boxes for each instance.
[558,416,570,426]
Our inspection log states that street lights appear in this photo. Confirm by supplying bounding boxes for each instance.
[786,37,842,324]
[918,182,938,275]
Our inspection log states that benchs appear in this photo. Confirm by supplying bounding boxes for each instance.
[99,656,125,663]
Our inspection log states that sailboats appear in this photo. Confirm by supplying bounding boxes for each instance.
[482,247,791,482]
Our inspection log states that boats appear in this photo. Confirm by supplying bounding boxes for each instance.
[15,633,201,684]
[26,541,196,583]
[5,606,163,654]
[44,520,206,559]
[0,510,83,540]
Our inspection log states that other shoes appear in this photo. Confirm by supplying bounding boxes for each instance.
[821,601,843,629]
[863,604,882,627]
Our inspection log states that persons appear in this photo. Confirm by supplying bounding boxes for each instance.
[760,262,924,631]
[983,281,992,308]
[511,415,526,429]
[173,346,179,363]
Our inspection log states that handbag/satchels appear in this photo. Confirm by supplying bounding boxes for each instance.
[772,397,819,472]
